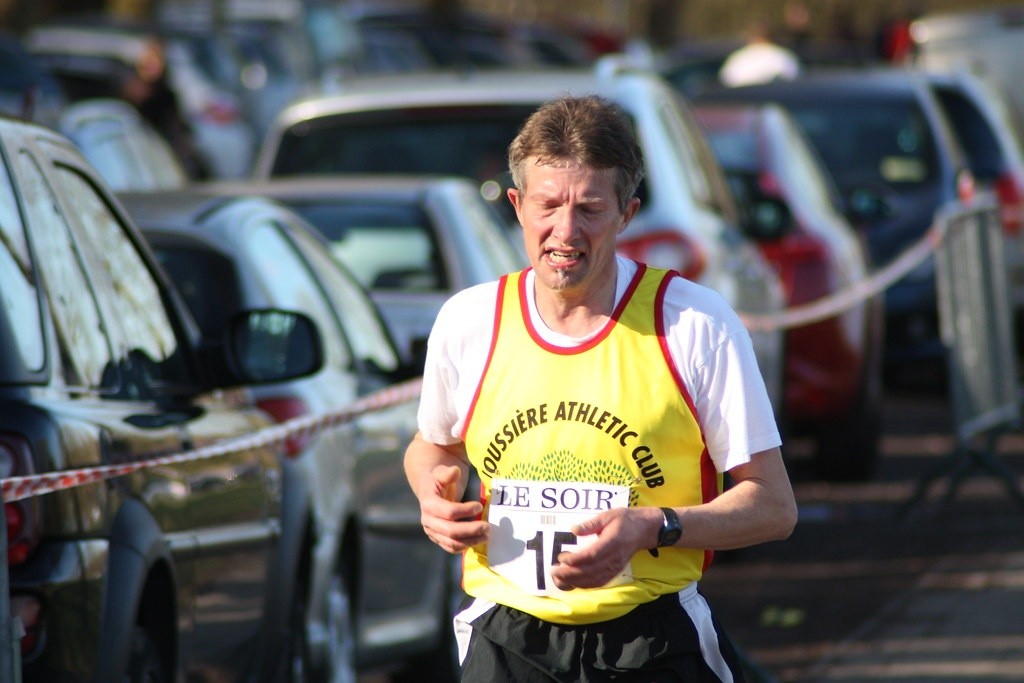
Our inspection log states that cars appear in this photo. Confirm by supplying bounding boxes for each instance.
[0,1,1024,683]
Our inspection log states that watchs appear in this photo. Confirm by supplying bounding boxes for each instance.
[649,507,682,558]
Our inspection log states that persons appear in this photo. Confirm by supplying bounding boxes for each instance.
[405,97,797,683]
